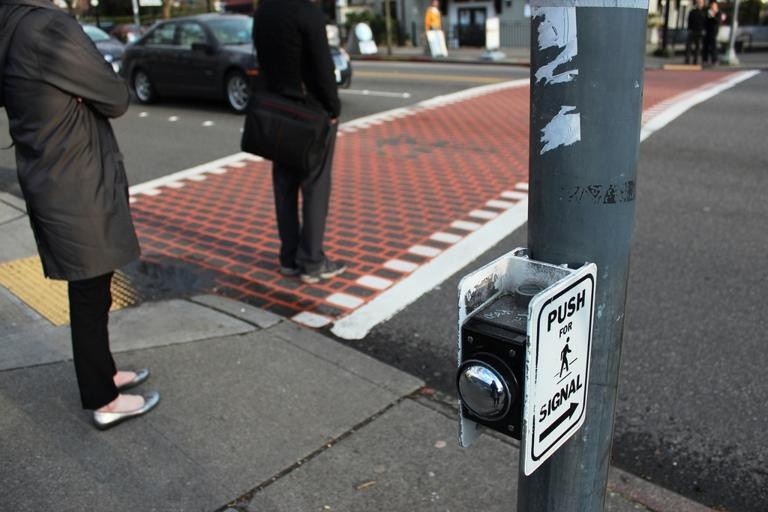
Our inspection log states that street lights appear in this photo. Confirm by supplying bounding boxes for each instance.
[90,0,100,30]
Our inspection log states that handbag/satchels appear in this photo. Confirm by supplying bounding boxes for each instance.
[241,90,324,170]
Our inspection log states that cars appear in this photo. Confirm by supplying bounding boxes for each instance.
[323,23,351,90]
[125,15,351,115]
[109,24,147,45]
[81,25,127,77]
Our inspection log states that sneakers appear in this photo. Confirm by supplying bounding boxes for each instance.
[281,258,348,284]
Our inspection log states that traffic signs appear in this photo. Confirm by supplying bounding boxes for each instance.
[522,262,597,484]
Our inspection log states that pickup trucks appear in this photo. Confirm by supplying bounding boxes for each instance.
[715,15,768,53]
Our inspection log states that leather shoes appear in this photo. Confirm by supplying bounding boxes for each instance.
[91,388,160,430]
[115,367,148,390]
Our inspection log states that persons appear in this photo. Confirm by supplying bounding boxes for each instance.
[682,1,704,66]
[703,2,722,67]
[0,1,161,432]
[238,1,348,284]
[425,1,444,33]
